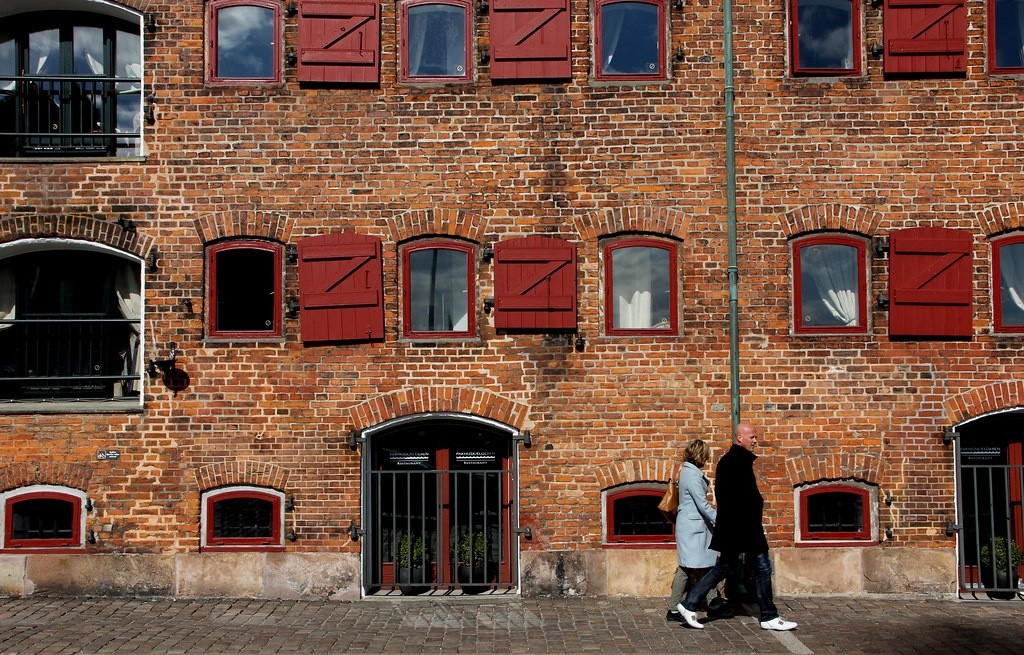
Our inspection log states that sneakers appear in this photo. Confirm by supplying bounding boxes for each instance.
[676,603,704,629]
[708,606,733,617]
[667,611,686,622]
[760,616,798,631]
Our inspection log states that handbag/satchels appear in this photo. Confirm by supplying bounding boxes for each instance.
[657,462,682,512]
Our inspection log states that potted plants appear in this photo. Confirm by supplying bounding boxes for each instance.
[980,537,1022,600]
[452,530,496,596]
[393,532,432,595]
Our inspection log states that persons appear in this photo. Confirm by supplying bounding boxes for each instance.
[665,438,729,623]
[676,421,800,633]
